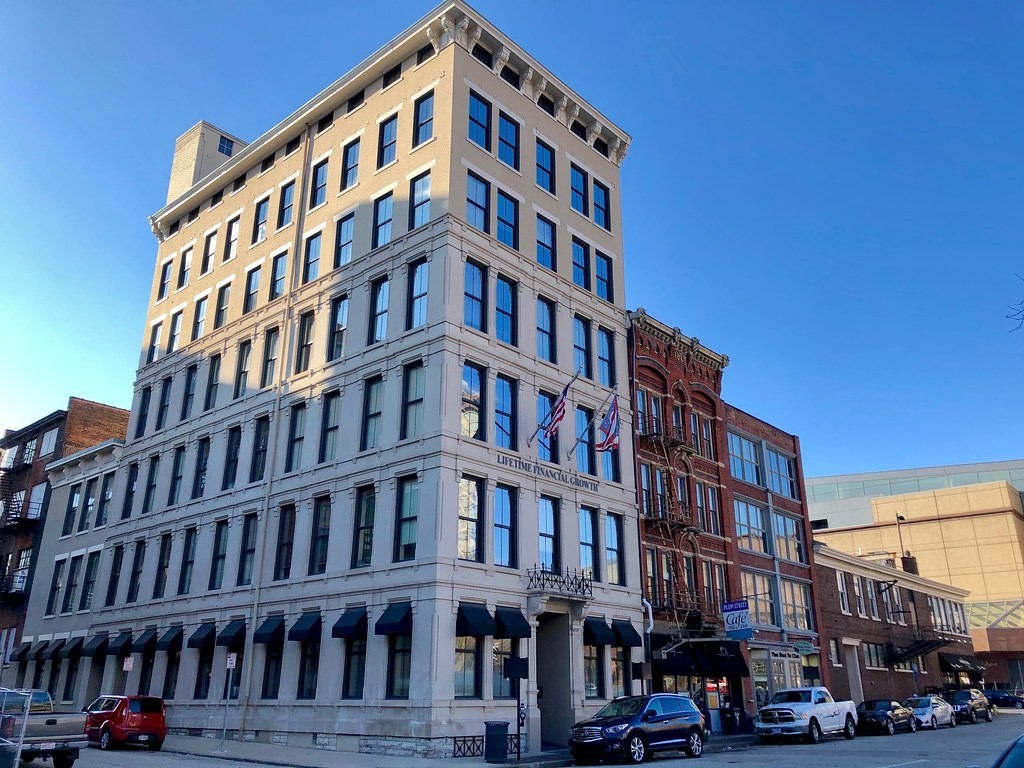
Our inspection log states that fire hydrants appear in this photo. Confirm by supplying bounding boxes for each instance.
[992,703,999,717]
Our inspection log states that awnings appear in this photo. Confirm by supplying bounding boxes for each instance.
[216,619,246,646]
[8,629,157,662]
[186,622,215,648]
[375,601,412,636]
[662,634,751,680]
[612,619,643,649]
[252,616,285,643]
[288,612,322,641]
[332,607,369,638]
[493,606,532,640]
[582,616,615,647]
[155,626,183,651]
[938,651,987,676]
[453,601,498,637]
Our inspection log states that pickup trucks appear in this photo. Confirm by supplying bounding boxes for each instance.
[0,686,91,767]
[755,686,858,744]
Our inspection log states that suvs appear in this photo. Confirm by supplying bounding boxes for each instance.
[564,691,711,762]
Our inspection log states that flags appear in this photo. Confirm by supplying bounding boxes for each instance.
[593,393,622,453]
[543,382,567,439]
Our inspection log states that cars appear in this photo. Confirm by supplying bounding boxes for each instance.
[854,698,918,735]
[950,687,993,724]
[980,689,1024,709]
[79,693,167,751]
[904,695,957,728]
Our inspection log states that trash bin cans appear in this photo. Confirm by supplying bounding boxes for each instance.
[484,721,510,764]
